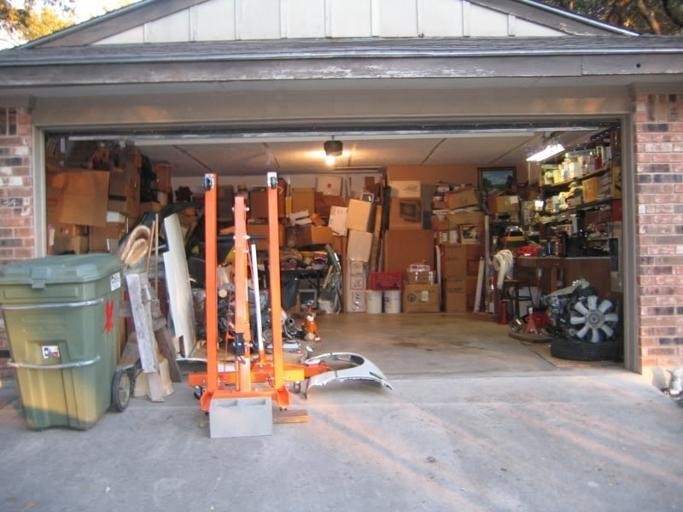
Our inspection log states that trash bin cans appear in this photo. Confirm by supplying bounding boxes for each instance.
[1,253,131,431]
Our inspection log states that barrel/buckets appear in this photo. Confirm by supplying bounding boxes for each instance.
[365,290,382,314]
[384,289,401,314]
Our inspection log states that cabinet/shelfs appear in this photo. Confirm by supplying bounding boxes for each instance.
[537,127,620,244]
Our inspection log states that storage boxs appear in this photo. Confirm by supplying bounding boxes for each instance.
[212,179,483,315]
[51,145,177,258]
[489,197,520,211]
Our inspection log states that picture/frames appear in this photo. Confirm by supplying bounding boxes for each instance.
[476,165,517,196]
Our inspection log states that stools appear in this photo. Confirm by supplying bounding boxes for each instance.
[501,279,532,327]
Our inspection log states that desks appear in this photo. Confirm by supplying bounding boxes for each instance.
[513,255,614,308]
